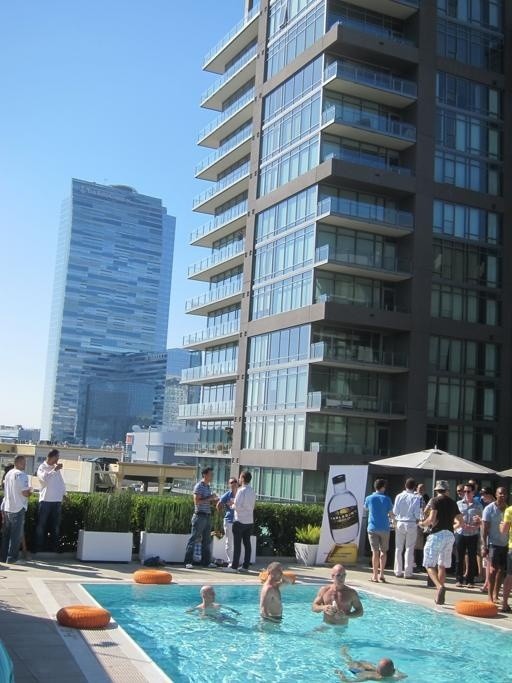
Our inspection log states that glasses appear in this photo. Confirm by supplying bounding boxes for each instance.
[334,573,347,578]
[463,489,471,494]
[230,480,237,484]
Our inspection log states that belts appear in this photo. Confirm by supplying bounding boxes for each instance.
[194,511,211,516]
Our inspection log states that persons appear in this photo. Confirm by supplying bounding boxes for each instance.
[222,471,256,573]
[392,478,421,579]
[184,584,241,624]
[334,646,409,683]
[184,467,218,569]
[414,478,512,614]
[364,479,393,583]
[311,564,364,637]
[259,561,283,633]
[215,477,239,568]
[30,448,65,553]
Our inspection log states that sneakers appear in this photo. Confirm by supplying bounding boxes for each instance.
[185,563,193,568]
[6,559,27,565]
[207,562,218,568]
[238,567,249,572]
[224,567,237,573]
[21,550,32,560]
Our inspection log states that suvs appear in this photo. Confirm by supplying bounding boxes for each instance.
[89,457,118,470]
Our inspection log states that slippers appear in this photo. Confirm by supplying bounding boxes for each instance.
[368,574,512,614]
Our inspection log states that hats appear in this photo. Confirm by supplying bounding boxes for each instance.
[433,480,450,490]
[480,486,494,495]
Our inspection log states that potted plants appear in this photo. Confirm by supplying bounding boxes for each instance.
[292,520,321,566]
[75,491,140,562]
[138,496,196,563]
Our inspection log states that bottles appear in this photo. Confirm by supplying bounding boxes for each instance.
[332,601,337,613]
[481,557,487,568]
[327,474,361,544]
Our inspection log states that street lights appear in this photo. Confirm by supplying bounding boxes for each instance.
[146,425,152,464]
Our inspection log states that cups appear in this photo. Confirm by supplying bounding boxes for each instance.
[230,497,235,503]
[211,490,216,496]
[420,512,424,519]
[57,463,62,469]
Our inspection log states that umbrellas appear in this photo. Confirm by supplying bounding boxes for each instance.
[369,445,499,498]
[495,469,512,478]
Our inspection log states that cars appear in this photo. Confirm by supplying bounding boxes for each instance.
[130,483,141,491]
